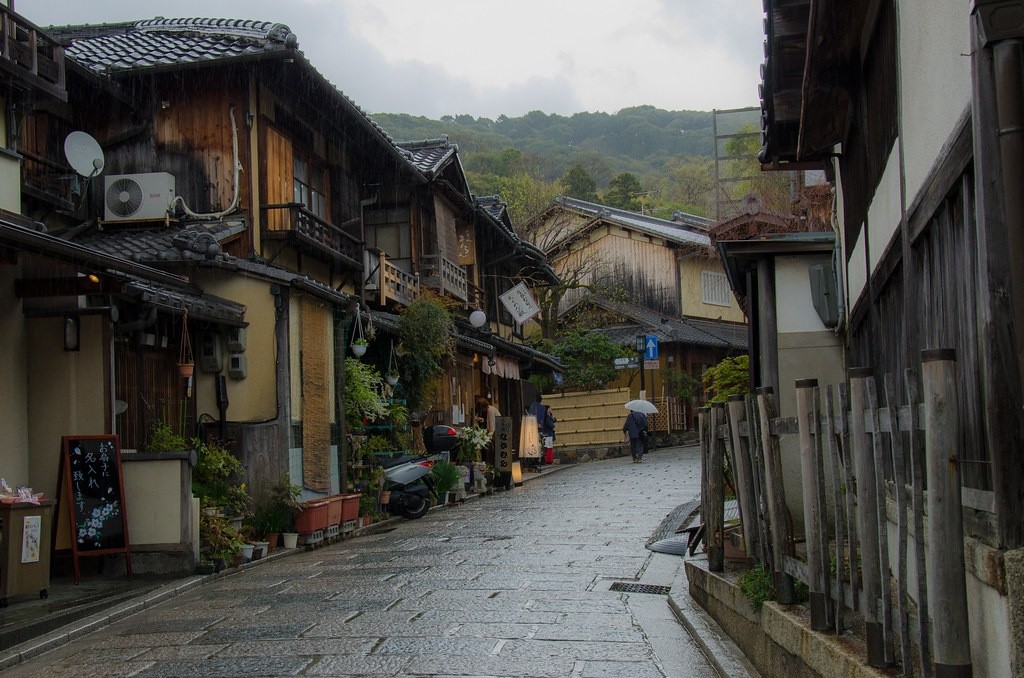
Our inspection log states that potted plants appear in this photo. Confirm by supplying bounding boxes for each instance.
[145,418,299,575]
[343,337,501,527]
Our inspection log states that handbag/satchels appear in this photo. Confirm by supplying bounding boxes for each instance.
[638,430,648,440]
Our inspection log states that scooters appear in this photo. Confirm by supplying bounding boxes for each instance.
[347,424,457,520]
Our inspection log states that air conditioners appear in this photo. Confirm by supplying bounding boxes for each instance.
[105,172,176,222]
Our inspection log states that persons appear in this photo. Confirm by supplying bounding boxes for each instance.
[528,395,557,464]
[623,410,648,462]
[475,398,501,432]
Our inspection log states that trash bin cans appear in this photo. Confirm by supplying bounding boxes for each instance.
[0,499,58,608]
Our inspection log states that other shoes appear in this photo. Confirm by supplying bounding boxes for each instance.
[637,458,642,463]
[633,458,637,463]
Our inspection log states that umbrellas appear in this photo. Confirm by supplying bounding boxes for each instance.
[625,400,658,413]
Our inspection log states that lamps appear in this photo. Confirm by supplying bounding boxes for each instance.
[469,306,486,328]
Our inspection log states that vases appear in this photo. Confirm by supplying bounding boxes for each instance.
[263,533,279,549]
[252,542,269,558]
[253,548,263,560]
[352,345,366,356]
[176,362,195,377]
[198,565,215,574]
[387,376,399,385]
[292,494,363,535]
[232,554,243,567]
[241,545,254,560]
[207,558,223,573]
[282,533,298,548]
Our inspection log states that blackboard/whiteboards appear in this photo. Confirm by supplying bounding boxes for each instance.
[63,435,129,555]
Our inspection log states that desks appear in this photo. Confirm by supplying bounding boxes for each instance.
[0,499,57,609]
